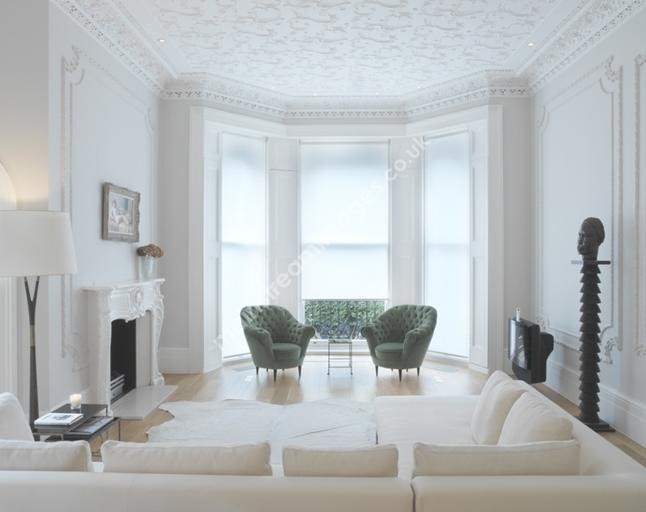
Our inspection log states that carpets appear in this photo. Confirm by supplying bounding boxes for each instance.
[148,399,377,464]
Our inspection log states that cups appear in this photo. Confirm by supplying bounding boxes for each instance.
[70,395,81,411]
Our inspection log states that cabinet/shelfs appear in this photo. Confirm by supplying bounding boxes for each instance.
[82,278,165,417]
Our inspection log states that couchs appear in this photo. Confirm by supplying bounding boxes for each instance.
[0,392,414,512]
[241,305,315,384]
[361,304,437,381]
[373,370,646,512]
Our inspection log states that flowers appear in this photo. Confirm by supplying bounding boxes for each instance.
[138,244,164,257]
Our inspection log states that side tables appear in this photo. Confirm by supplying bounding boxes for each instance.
[32,402,108,441]
[65,416,120,457]
[328,338,352,375]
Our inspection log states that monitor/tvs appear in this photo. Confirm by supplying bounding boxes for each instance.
[508,317,555,385]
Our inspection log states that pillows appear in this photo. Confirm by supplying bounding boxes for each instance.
[469,370,527,445]
[412,442,581,476]
[283,445,399,477]
[0,440,93,472]
[497,392,572,445]
[100,440,276,475]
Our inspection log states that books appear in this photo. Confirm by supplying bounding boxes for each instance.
[34,413,84,425]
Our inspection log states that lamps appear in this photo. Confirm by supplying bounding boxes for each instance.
[0,210,79,440]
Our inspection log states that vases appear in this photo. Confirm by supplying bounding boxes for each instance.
[143,256,155,280]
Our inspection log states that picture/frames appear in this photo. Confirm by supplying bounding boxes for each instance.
[102,183,140,242]
